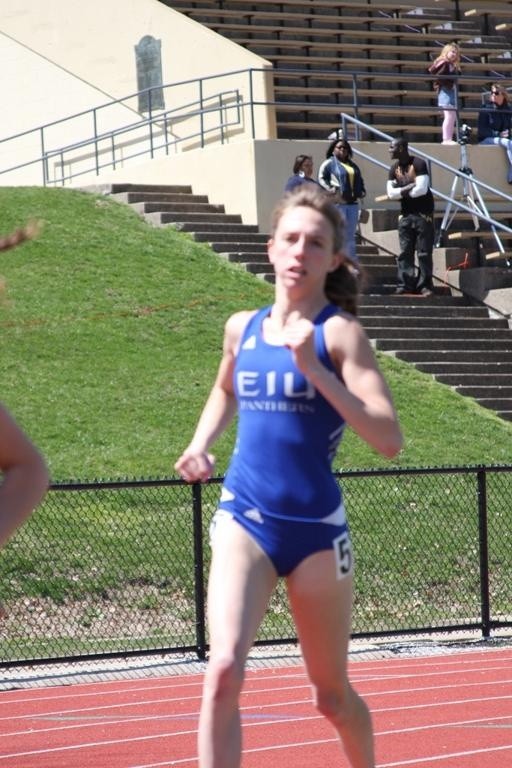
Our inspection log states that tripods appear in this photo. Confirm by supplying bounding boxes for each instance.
[434,145,512,267]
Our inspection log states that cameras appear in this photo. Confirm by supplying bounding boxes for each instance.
[458,124,473,145]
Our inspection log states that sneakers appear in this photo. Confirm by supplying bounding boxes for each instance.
[395,287,435,296]
[441,140,458,145]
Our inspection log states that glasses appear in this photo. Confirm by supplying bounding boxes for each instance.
[491,91,501,96]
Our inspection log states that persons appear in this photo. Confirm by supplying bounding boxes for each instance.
[373,137,436,297]
[283,154,336,198]
[1,398,49,548]
[476,85,512,185]
[327,113,359,142]
[427,41,463,147]
[318,138,367,275]
[171,186,405,768]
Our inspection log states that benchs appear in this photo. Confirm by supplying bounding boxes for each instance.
[160,0,511,145]
[433,193,512,260]
